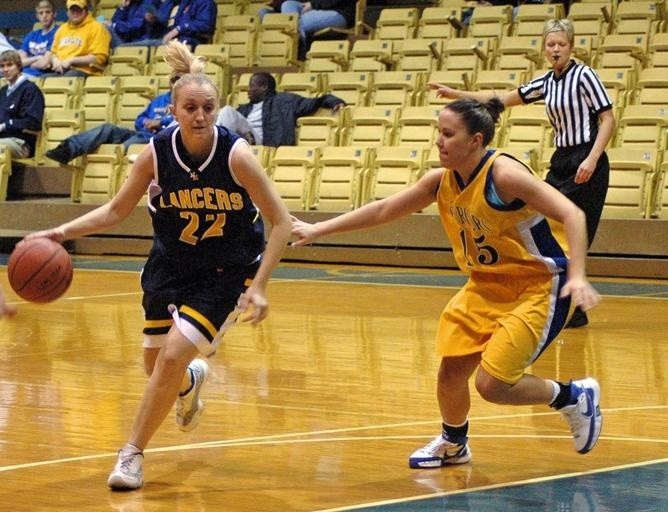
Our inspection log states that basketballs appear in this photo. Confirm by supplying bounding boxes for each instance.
[8,237,73,303]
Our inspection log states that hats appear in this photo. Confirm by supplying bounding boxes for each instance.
[66,0,89,10]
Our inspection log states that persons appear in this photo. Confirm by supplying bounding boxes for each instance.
[18,1,218,78]
[214,73,348,147]
[15,38,294,491]
[267,0,356,60]
[427,18,616,329]
[0,51,46,157]
[288,97,603,469]
[45,76,180,165]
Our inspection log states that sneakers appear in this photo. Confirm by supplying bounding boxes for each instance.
[107,449,145,488]
[408,433,472,468]
[46,145,68,165]
[555,377,603,454]
[176,358,210,432]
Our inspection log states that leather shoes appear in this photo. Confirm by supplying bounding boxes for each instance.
[564,305,589,329]
